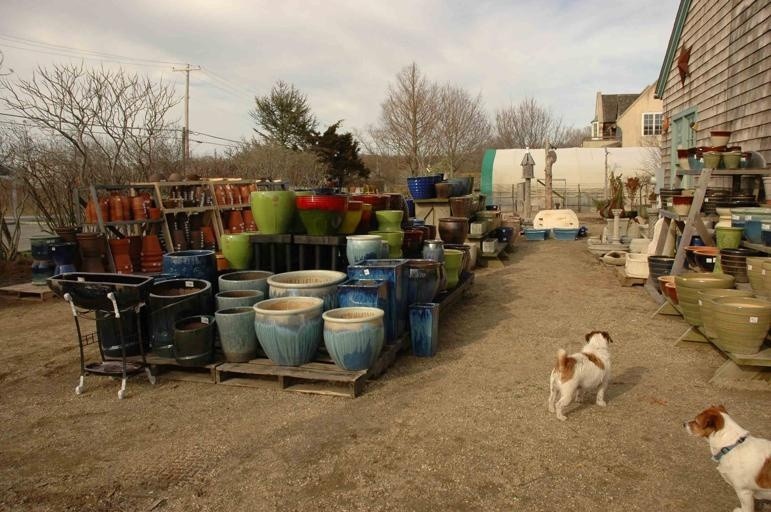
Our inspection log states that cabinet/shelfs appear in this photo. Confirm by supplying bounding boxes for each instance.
[644,168,770,392]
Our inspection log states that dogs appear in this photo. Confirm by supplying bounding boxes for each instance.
[548,330,614,422]
[681,404,771,512]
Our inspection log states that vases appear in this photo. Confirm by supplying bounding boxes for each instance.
[251,296,324,367]
[524,228,579,243]
[215,307,258,363]
[322,306,385,369]
[408,302,440,358]
[587,130,770,339]
[27,172,523,343]
[172,315,215,365]
[712,296,770,352]
